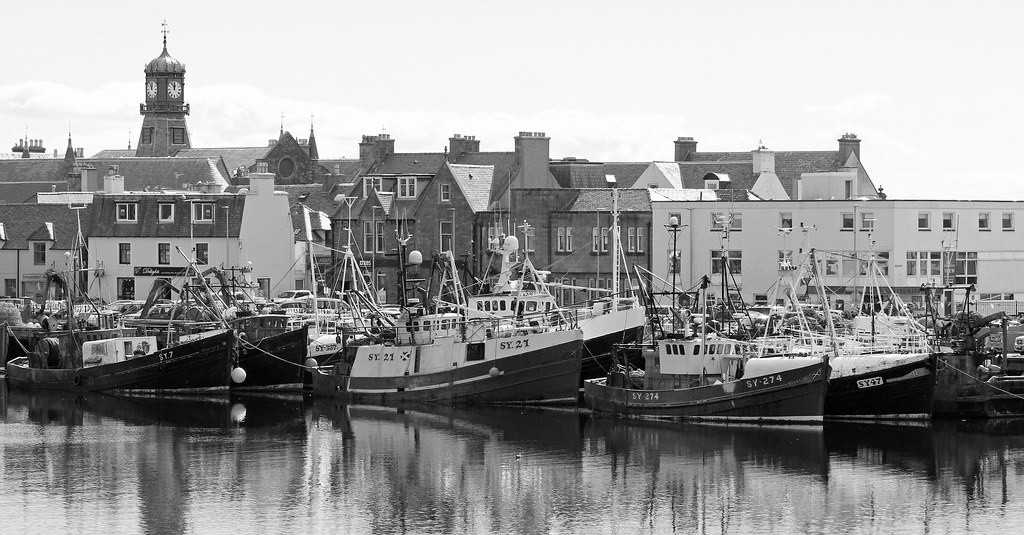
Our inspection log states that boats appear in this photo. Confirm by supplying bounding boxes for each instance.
[1,195,1024,444]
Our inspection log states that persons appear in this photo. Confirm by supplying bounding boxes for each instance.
[133,345,146,359]
